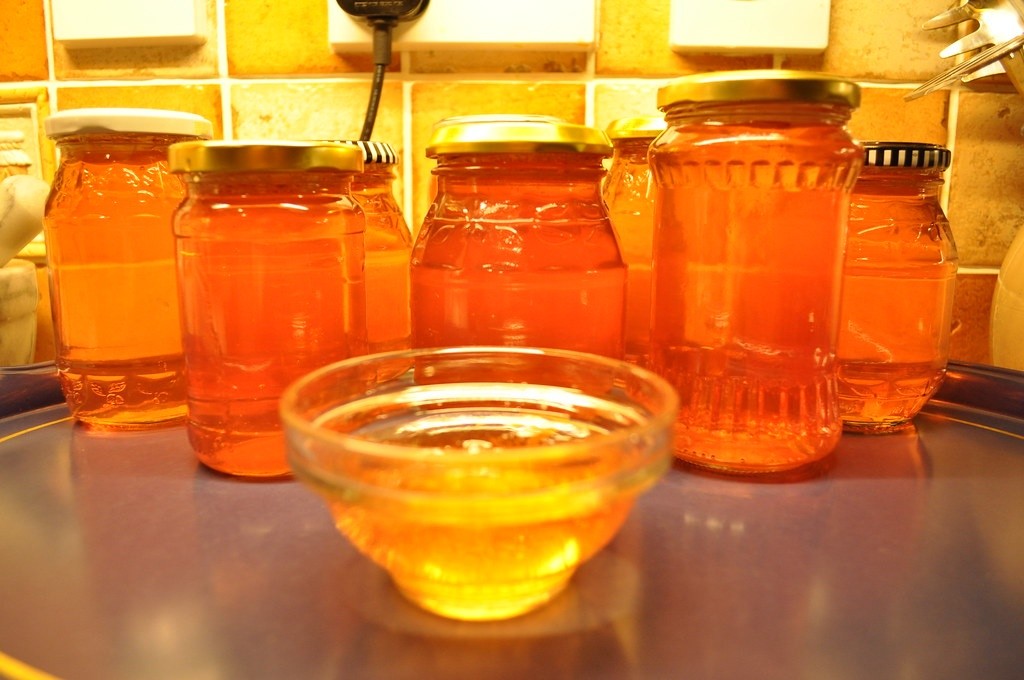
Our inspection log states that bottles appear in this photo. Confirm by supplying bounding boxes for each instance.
[40,106,413,481]
[407,70,961,478]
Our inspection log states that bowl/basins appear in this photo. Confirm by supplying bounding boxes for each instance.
[276,344,683,623]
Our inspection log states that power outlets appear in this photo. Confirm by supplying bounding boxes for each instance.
[329,0,599,53]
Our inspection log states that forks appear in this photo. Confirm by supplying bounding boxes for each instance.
[921,0,1024,85]
